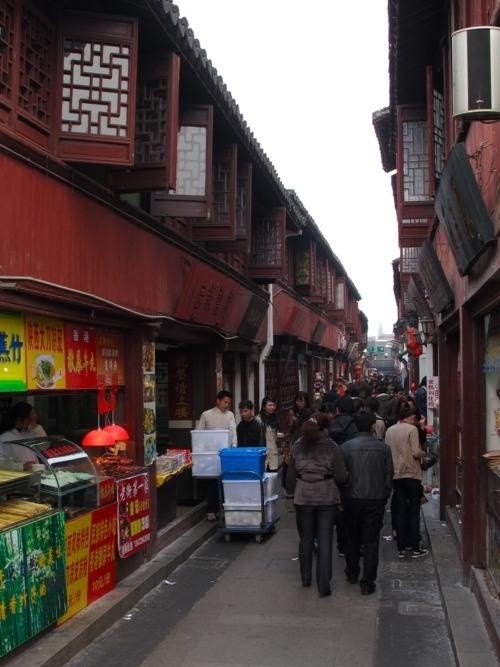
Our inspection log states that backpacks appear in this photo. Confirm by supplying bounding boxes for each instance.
[329,418,354,444]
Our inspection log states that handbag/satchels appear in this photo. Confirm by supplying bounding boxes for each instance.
[284,459,296,492]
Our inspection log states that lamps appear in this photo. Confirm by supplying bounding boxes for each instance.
[82,387,130,447]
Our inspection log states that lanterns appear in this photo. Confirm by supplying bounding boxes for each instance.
[413,344,423,356]
[406,339,418,351]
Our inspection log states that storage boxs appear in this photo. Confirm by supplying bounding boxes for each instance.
[190,429,281,528]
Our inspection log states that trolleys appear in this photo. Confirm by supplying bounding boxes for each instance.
[218,465,283,546]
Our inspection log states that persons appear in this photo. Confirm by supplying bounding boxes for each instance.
[384,401,431,560]
[193,389,238,520]
[0,402,48,474]
[285,412,352,593]
[340,408,393,593]
[278,368,432,499]
[235,402,264,446]
[256,398,281,472]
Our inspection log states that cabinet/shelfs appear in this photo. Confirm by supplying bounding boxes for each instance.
[2,436,99,518]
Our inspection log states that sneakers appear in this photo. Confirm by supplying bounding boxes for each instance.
[411,549,429,559]
[396,551,406,558]
[207,513,216,521]
[318,587,331,597]
[361,584,376,595]
